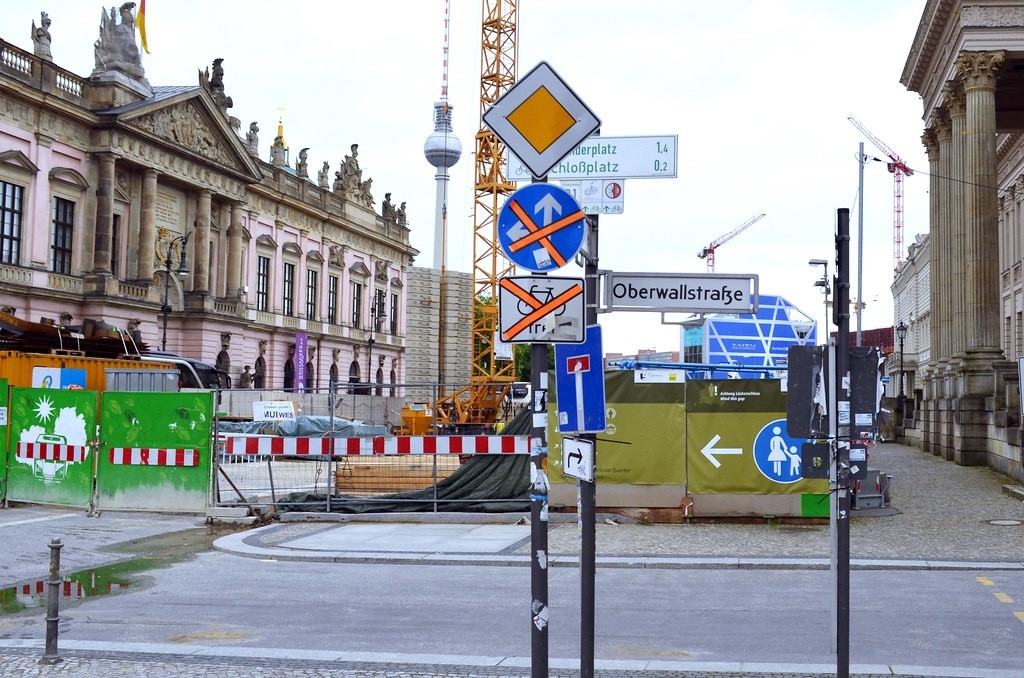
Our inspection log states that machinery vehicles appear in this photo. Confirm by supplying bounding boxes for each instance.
[393,1,522,436]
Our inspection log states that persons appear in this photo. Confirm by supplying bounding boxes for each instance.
[272,137,284,160]
[398,202,406,225]
[240,366,257,387]
[320,161,329,185]
[298,148,309,176]
[333,147,377,206]
[384,193,395,219]
[37,12,51,43]
[249,122,259,145]
[156,103,203,146]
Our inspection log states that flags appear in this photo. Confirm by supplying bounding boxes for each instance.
[134,0,151,54]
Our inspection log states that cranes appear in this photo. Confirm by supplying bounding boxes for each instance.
[697,213,766,272]
[844,114,916,273]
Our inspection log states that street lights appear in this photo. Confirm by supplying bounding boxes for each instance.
[895,320,907,413]
[368,296,388,384]
[808,257,829,343]
[162,236,189,350]
